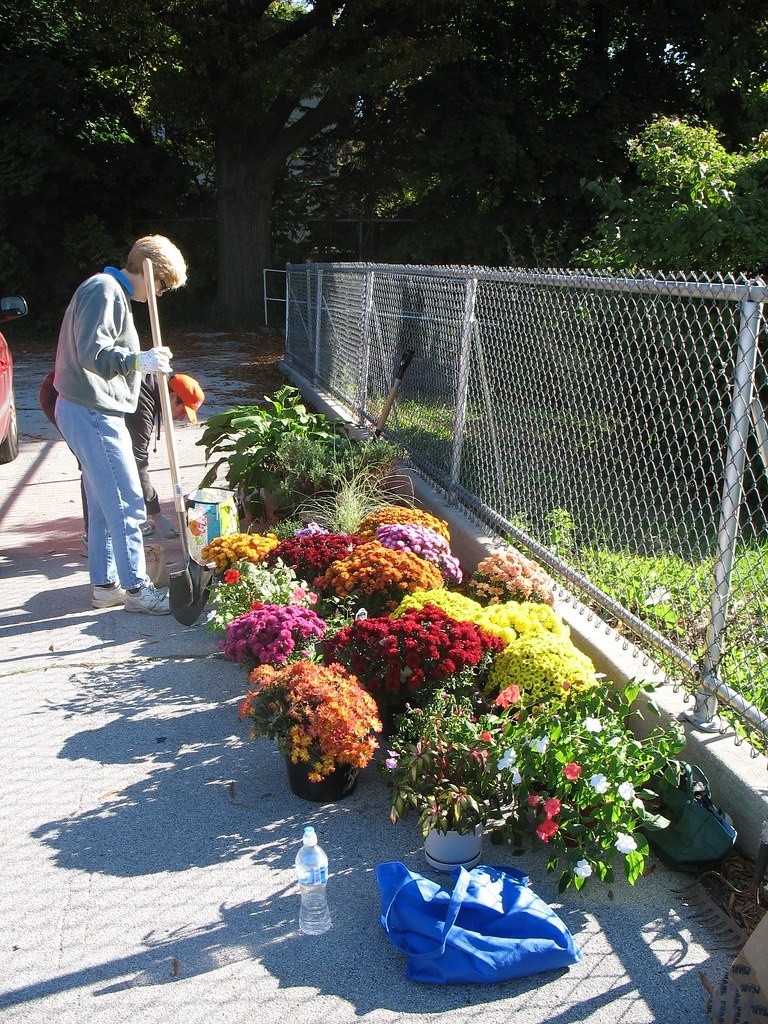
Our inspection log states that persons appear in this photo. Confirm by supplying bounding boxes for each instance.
[52,234,188,617]
[38,368,205,558]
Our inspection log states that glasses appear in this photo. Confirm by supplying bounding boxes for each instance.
[157,272,170,294]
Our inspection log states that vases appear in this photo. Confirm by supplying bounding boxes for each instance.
[424,823,484,872]
[530,785,604,847]
[285,754,358,803]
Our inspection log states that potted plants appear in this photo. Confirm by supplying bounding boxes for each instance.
[195,383,356,522]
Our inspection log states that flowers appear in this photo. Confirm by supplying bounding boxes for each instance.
[196,504,686,890]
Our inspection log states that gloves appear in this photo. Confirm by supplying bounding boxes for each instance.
[138,346,174,373]
[152,515,175,539]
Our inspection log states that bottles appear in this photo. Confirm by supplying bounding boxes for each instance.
[295,826,331,935]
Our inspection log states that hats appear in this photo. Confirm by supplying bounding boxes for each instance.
[168,373,205,426]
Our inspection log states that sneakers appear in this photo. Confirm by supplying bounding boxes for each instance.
[139,519,155,536]
[124,583,171,615]
[80,534,89,556]
[91,579,125,608]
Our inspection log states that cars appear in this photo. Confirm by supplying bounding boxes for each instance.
[0,294,28,464]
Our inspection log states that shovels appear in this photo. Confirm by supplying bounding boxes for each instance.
[142,257,216,627]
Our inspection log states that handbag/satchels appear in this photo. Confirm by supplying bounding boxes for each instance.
[637,757,737,873]
[375,862,582,984]
